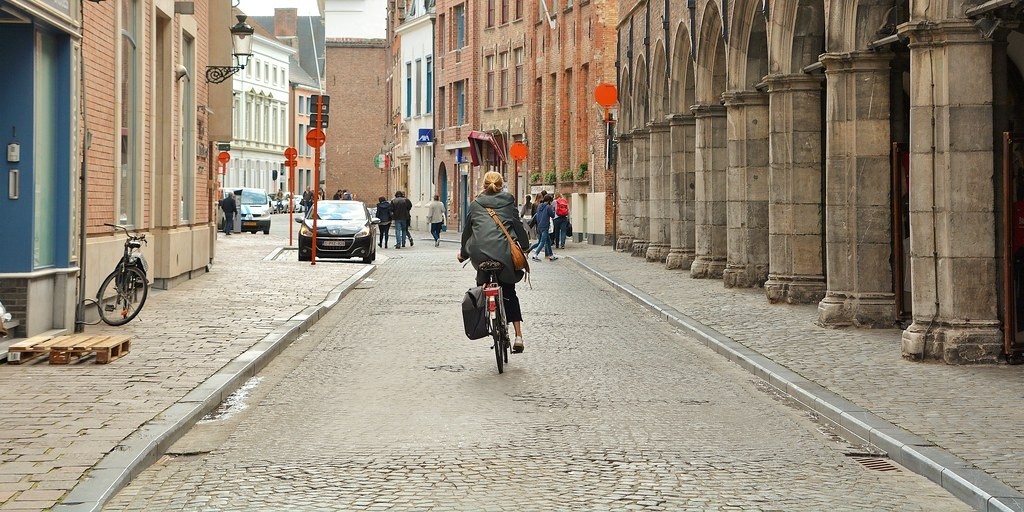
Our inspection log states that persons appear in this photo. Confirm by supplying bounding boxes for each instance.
[221,191,238,235]
[376,191,414,249]
[303,187,325,217]
[427,195,446,247]
[520,190,570,262]
[277,189,284,209]
[333,189,352,200]
[456,171,530,351]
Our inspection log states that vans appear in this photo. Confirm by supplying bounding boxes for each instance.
[215,187,308,235]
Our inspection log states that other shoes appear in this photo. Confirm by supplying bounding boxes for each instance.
[524,250,529,258]
[561,246,564,249]
[394,245,400,249]
[513,335,525,351]
[550,253,558,261]
[402,245,405,247]
[545,256,551,260]
[410,237,413,246]
[435,238,440,247]
[532,254,541,262]
[385,246,388,248]
[378,243,382,247]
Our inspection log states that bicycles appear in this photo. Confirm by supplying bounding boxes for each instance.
[96,223,149,326]
[459,255,525,374]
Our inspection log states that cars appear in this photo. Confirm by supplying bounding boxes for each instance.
[295,199,382,264]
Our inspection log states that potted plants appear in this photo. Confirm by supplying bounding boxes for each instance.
[528,162,588,185]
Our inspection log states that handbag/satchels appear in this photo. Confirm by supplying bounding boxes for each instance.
[114,253,148,288]
[510,241,530,274]
[462,285,489,340]
[566,219,573,237]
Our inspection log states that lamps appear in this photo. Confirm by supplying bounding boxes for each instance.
[206,15,254,84]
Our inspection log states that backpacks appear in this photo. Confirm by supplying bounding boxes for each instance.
[555,198,569,217]
